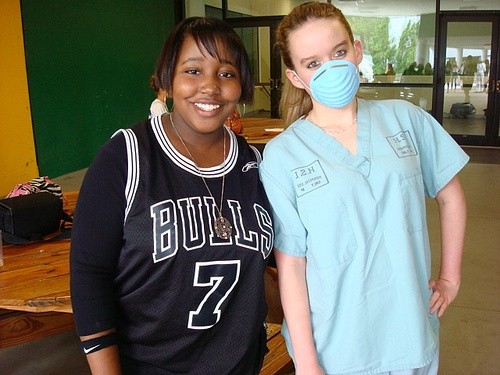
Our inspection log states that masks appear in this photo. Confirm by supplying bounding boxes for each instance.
[292,58,360,109]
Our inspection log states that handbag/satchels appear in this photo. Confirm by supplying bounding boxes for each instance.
[224,108,242,133]
[0,193,74,244]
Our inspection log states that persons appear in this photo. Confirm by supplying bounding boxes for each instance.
[258,0,471,375]
[69,16,285,375]
[400,62,434,110]
[444,55,490,103]
[385,63,395,84]
[359,72,368,83]
[148,75,170,119]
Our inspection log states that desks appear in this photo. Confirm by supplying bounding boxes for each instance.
[0,192,80,314]
[226,117,284,145]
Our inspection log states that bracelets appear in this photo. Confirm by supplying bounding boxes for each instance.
[80,332,117,355]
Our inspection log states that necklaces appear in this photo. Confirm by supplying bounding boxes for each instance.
[308,109,358,134]
[170,112,232,239]
